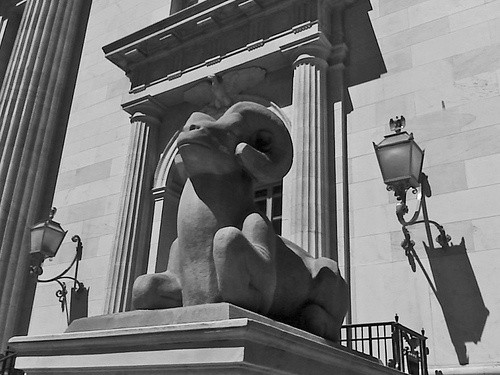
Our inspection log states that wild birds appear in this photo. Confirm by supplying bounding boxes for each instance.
[183,67,266,109]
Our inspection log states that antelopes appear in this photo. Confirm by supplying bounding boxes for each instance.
[130,101,352,343]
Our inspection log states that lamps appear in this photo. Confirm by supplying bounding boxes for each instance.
[370,114,453,275]
[22,205,83,313]
